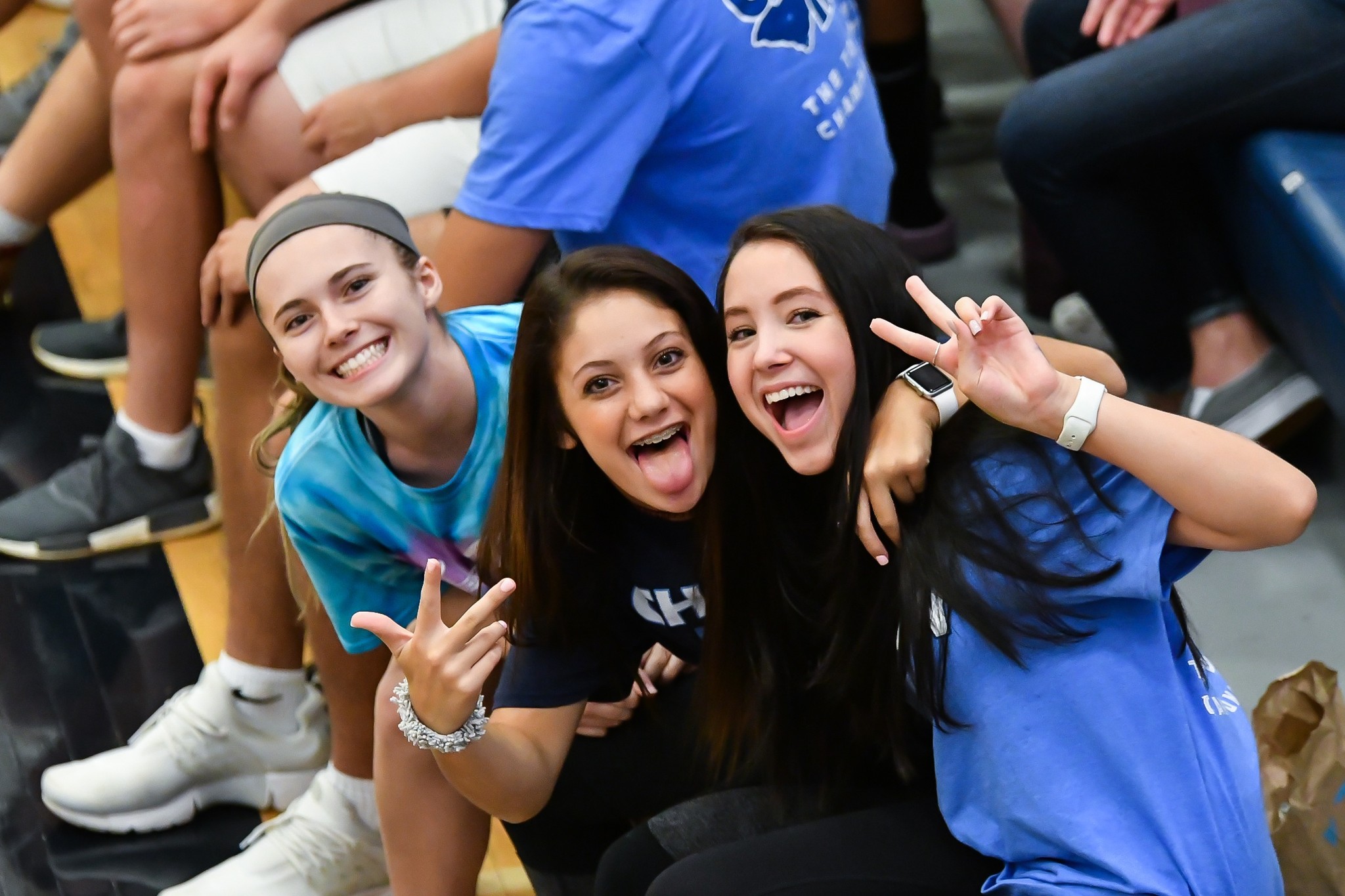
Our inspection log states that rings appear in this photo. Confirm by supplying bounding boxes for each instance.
[932,343,941,365]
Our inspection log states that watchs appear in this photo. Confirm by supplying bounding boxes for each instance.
[893,359,959,429]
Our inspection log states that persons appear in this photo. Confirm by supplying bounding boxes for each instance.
[0,0,1344,896]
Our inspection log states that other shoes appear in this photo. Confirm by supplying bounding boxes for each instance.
[889,203,959,263]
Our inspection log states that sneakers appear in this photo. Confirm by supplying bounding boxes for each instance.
[1179,345,1321,441]
[0,415,226,558]
[41,660,331,834]
[29,309,219,383]
[157,762,391,896]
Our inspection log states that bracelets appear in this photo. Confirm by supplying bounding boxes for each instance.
[1053,378,1106,453]
[389,677,489,754]
